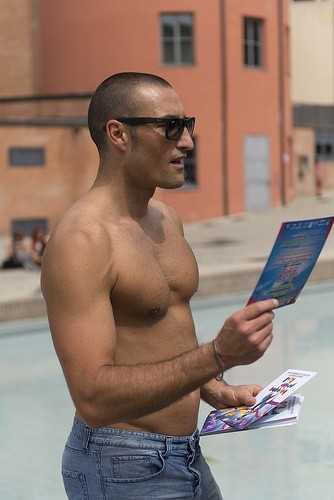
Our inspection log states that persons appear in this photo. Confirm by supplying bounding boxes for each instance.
[21,225,48,269]
[38,71,278,500]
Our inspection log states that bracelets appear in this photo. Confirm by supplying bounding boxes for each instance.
[210,339,225,383]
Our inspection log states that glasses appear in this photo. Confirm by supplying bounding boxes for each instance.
[118,116,197,141]
